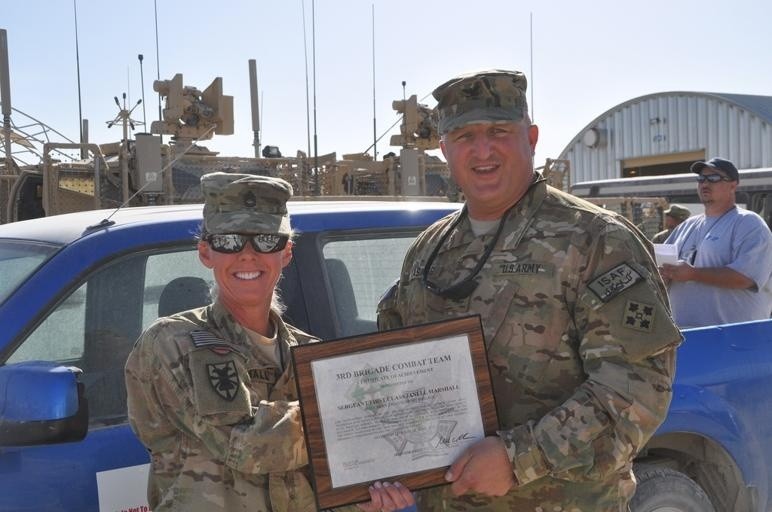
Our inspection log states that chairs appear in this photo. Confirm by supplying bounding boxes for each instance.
[158,277,212,317]
[325,259,379,337]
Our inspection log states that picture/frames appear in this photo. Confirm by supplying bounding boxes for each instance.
[291,314,501,511]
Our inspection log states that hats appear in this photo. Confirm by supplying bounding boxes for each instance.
[200,172,294,234]
[691,157,739,184]
[431,70,527,136]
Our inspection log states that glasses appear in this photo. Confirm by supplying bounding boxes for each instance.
[423,172,546,299]
[697,174,734,182]
[202,233,289,254]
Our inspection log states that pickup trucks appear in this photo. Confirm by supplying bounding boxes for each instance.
[0,193,771,512]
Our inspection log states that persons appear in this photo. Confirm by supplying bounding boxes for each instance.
[125,171,419,511]
[657,158,772,331]
[648,204,691,243]
[375,69,688,511]
